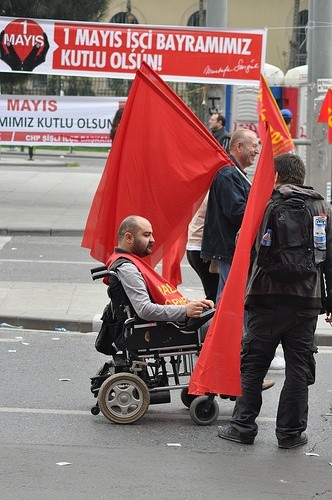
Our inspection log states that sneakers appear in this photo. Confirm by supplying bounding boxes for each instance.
[269,355,286,370]
[218,423,255,445]
[278,433,308,449]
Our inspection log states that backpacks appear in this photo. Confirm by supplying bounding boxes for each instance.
[257,189,327,282]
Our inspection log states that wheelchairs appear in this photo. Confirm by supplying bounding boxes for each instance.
[90,266,237,425]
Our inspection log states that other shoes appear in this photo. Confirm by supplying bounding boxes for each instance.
[262,378,275,390]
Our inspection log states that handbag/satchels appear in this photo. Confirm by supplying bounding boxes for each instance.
[94,303,128,355]
[209,257,219,273]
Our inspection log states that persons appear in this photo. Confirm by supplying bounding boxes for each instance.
[208,113,231,155]
[281,108,293,130]
[217,154,332,447]
[106,214,275,401]
[109,109,123,144]
[200,128,286,368]
[186,189,221,304]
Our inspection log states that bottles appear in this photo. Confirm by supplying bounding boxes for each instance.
[313,223,326,264]
[261,229,272,246]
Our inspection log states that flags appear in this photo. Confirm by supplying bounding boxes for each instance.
[187,121,275,397]
[317,90,332,144]
[258,75,292,154]
[79,60,236,290]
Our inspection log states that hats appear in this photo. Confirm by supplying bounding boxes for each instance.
[280,108,292,119]
